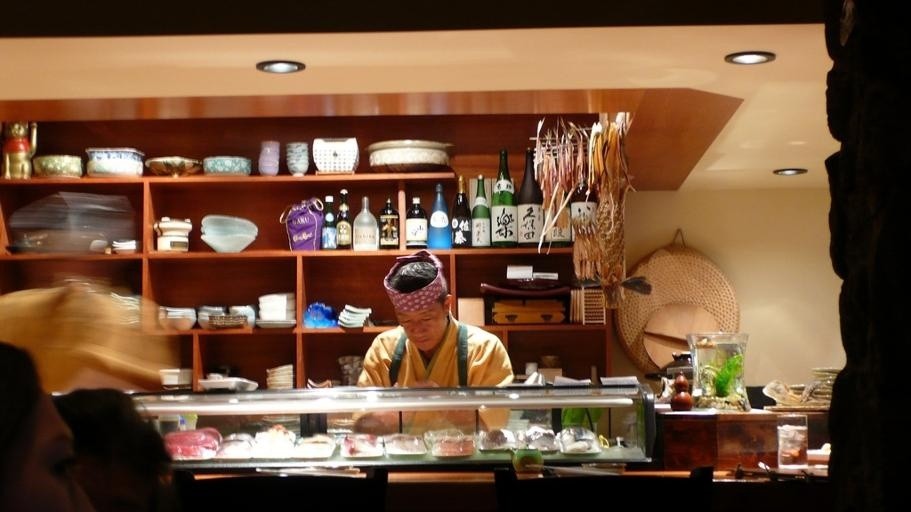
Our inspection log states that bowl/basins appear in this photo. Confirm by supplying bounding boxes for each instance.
[32,154,83,178]
[197,215,260,253]
[258,140,282,176]
[83,146,147,176]
[199,156,253,176]
[144,156,203,176]
[266,363,294,390]
[197,304,225,329]
[285,141,310,177]
[158,306,196,330]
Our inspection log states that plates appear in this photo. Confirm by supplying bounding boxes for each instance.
[384,433,420,457]
[216,438,252,461]
[167,448,214,463]
[112,238,140,254]
[342,435,384,458]
[251,435,292,460]
[294,438,337,459]
[253,319,297,328]
[107,292,141,328]
[775,363,841,408]
[513,431,554,453]
[421,435,474,460]
[555,429,601,454]
[197,376,259,391]
[474,432,513,451]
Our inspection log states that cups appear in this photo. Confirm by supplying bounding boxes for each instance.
[775,413,810,471]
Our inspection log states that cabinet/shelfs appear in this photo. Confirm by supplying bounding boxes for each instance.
[0,90,614,465]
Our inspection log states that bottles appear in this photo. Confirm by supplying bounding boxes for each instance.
[492,146,600,246]
[321,175,491,250]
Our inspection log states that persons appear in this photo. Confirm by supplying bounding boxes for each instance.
[2,192,179,398]
[0,343,95,512]
[352,250,515,434]
[51,387,174,512]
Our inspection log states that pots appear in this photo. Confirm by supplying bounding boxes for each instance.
[364,140,456,172]
